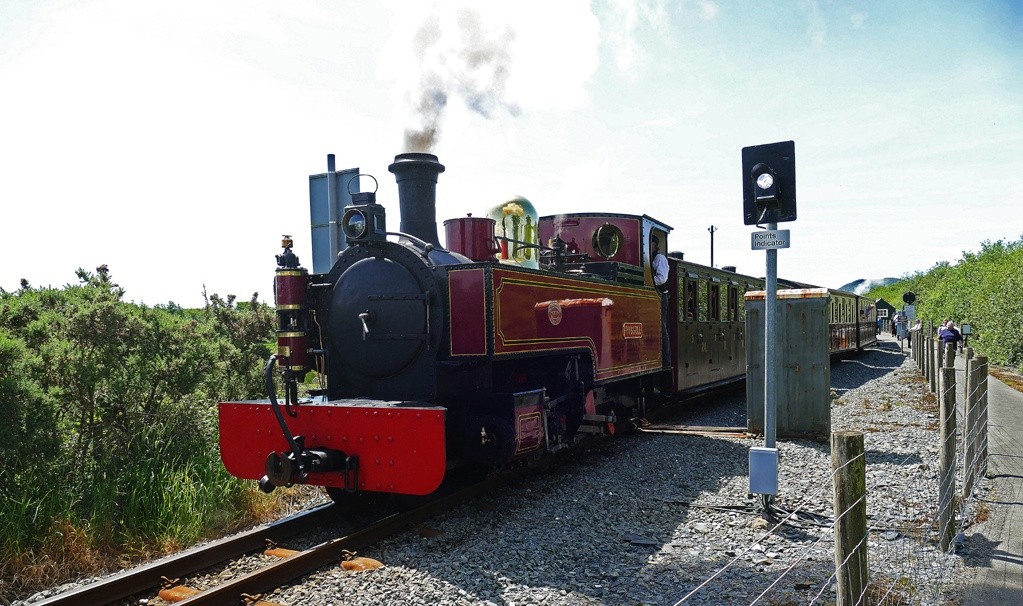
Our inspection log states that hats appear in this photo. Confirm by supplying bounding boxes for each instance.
[652,235,659,245]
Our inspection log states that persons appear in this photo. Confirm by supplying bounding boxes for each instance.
[877,315,882,337]
[652,236,672,370]
[937,318,963,361]
[891,310,902,337]
[910,319,922,333]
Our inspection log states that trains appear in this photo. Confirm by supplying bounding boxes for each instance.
[215,149,882,517]
